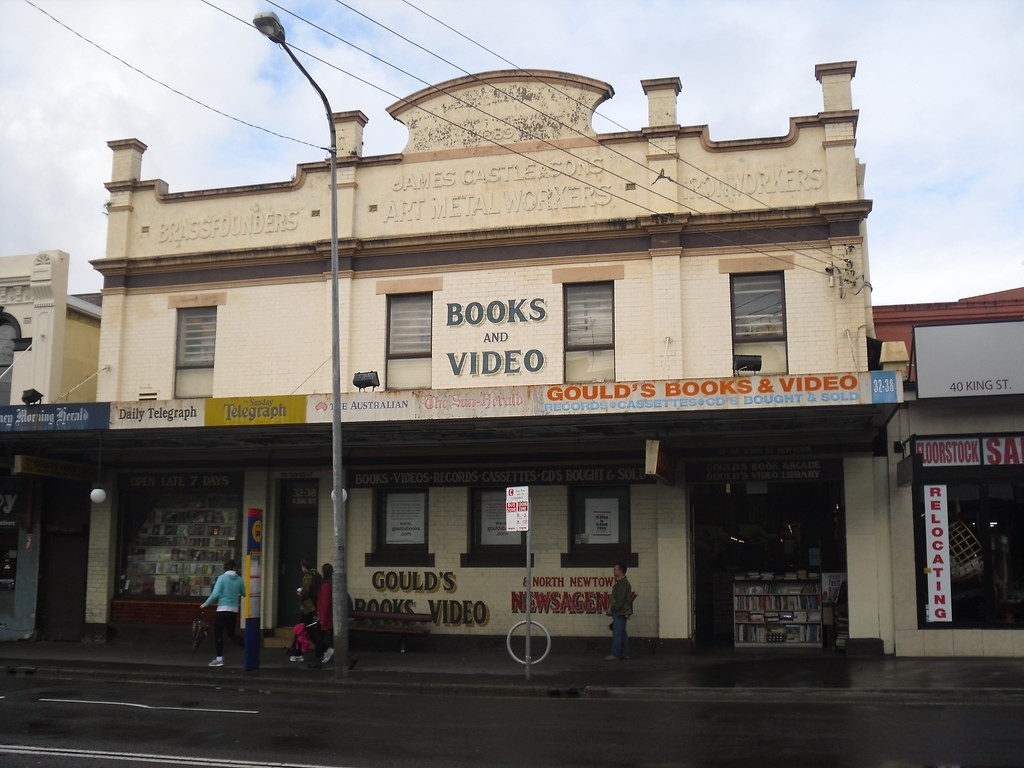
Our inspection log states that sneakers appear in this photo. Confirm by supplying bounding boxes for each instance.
[208,657,225,666]
[321,647,334,664]
[289,655,305,662]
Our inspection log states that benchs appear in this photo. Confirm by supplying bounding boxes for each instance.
[349,608,436,654]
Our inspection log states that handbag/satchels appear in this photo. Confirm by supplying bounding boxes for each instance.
[616,599,633,617]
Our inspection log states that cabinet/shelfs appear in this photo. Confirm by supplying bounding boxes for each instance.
[731,571,824,648]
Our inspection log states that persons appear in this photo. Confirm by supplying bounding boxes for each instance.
[290,560,334,663]
[317,563,333,631]
[604,561,631,660]
[199,558,246,667]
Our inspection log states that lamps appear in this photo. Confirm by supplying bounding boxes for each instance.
[90,430,107,505]
[21,388,43,406]
[732,353,763,378]
[352,370,380,393]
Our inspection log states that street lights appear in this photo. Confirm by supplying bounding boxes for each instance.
[252,8,354,683]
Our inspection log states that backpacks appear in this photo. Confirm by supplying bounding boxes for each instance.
[306,572,322,606]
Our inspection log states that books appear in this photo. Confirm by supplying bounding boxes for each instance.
[735,569,822,643]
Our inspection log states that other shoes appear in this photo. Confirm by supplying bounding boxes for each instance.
[605,655,619,660]
[349,657,359,670]
[622,652,630,660]
[306,662,322,669]
